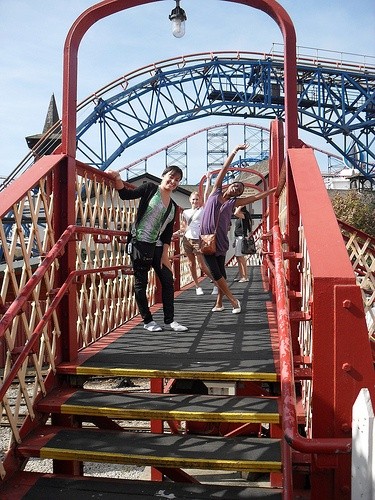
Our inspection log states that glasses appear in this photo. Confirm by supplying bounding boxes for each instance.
[192,199,198,200]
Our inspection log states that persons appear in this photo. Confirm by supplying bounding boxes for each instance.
[231,205,253,283]
[171,192,219,295]
[103,165,188,331]
[197,142,277,314]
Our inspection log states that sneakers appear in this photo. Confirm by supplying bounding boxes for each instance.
[144,321,162,332]
[164,320,188,332]
[212,286,219,295]
[196,287,203,295]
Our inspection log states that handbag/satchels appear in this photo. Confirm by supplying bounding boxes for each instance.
[199,234,216,254]
[130,240,156,263]
[241,240,256,254]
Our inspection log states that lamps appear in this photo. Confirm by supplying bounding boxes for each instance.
[169,0,187,38]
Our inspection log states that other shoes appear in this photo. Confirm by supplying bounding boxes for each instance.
[212,305,224,311]
[232,299,241,313]
[234,277,249,282]
[210,279,213,283]
